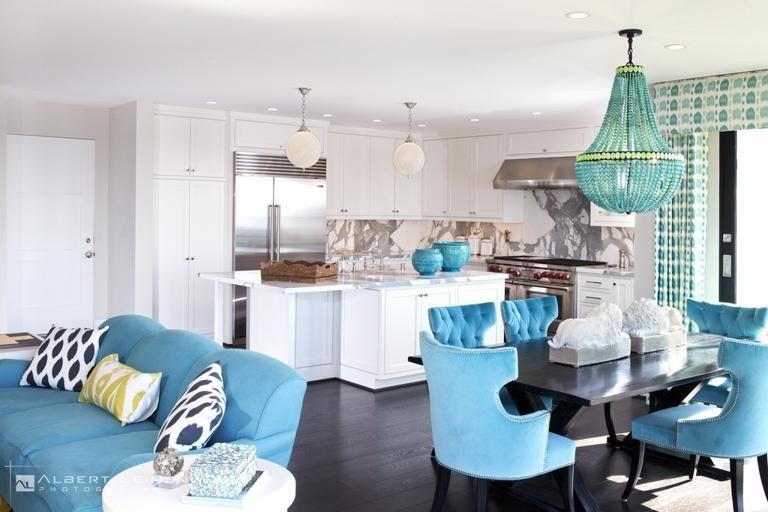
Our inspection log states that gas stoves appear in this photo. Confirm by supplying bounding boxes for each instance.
[485,254,607,282]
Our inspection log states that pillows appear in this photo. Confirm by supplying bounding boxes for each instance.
[15,323,107,394]
[155,358,226,452]
[76,350,163,430]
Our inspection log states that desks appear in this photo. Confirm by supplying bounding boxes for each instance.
[408,332,762,512]
[101,450,296,512]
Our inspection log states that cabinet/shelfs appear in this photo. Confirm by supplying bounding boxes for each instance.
[448,269,510,305]
[152,102,228,176]
[155,176,231,341]
[572,271,633,318]
[340,279,450,392]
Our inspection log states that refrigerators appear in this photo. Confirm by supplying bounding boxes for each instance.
[229,151,325,347]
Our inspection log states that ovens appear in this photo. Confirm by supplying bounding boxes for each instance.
[502,279,574,335]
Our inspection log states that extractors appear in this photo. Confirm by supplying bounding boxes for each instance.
[493,158,578,190]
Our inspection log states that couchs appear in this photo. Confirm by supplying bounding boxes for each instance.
[0,314,307,512]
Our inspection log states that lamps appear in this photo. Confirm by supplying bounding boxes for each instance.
[285,88,322,173]
[574,28,687,218]
[393,102,427,174]
